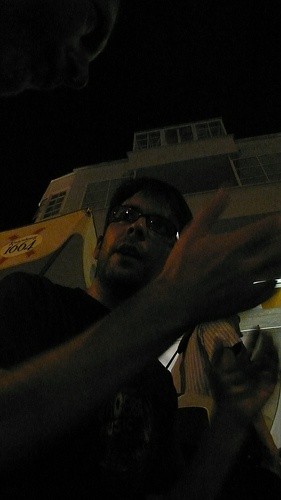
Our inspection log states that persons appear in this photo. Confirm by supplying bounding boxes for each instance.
[1,0,123,96]
[1,180,280,500]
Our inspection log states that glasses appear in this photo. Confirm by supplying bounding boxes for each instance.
[109,207,181,242]
